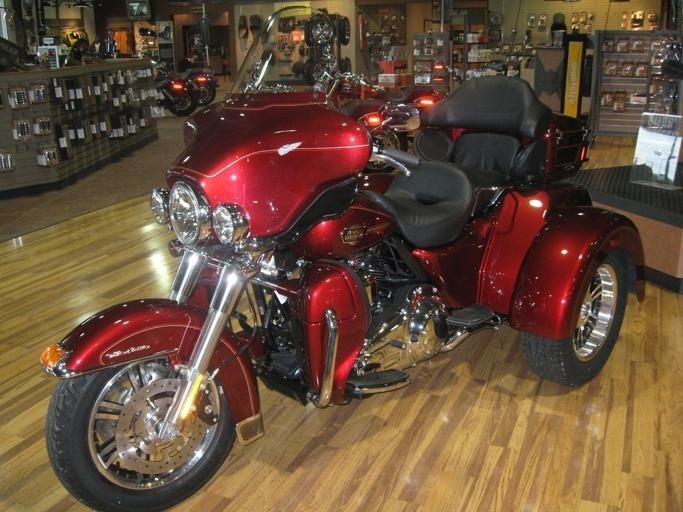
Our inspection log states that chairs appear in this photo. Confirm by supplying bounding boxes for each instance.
[367,74,553,247]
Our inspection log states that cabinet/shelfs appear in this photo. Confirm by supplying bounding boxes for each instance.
[447,39,543,98]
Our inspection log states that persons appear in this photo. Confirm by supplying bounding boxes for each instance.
[220,47,232,82]
[181,38,207,70]
[58,28,118,67]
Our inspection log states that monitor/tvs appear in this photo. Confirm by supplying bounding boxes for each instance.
[126,0,152,21]
